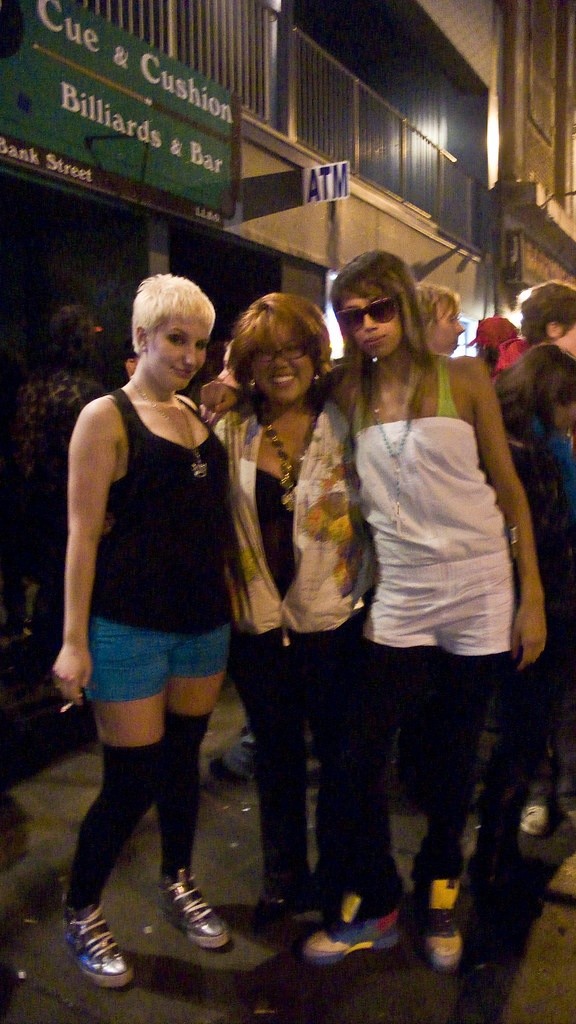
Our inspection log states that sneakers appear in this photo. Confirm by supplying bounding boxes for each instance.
[62,894,135,989]
[414,878,465,974]
[301,893,400,964]
[158,868,232,949]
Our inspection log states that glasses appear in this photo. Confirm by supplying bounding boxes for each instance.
[336,295,399,336]
[252,344,307,364]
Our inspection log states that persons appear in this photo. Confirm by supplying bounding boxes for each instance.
[1,246,576,989]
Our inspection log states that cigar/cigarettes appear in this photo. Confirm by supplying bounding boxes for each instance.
[61,694,82,712]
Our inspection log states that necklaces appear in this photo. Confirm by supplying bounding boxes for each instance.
[263,418,296,511]
[135,384,207,478]
[373,409,412,516]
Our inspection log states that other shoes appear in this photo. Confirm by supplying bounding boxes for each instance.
[518,805,548,837]
[253,891,294,934]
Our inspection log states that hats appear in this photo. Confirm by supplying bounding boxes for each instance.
[468,314,519,353]
